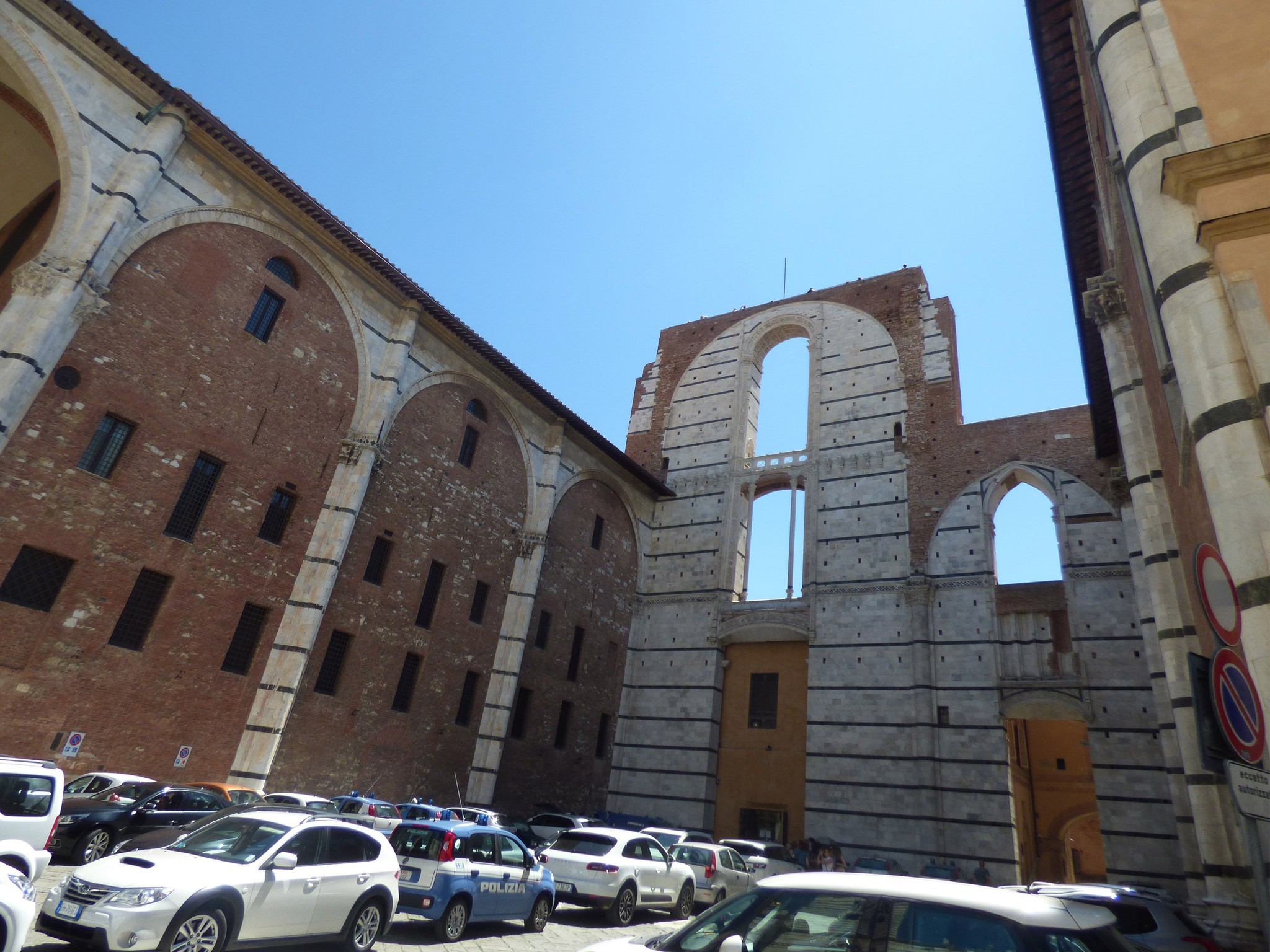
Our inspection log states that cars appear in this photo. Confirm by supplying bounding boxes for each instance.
[718,838,806,882]
[33,808,402,952]
[1,861,37,952]
[0,757,715,884]
[387,809,556,943]
[533,827,697,929]
[572,871,1141,952]
[997,880,1229,952]
[667,841,757,906]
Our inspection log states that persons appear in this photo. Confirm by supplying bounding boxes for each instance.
[280,837,312,866]
[785,837,847,872]
[158,796,169,810]
[974,860,990,885]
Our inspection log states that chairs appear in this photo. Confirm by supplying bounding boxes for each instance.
[406,808,417,820]
[347,802,356,813]
[473,851,491,863]
[688,850,724,868]
[249,826,285,853]
[828,912,863,939]
[626,841,640,859]
[792,919,810,934]
[421,811,428,819]
[158,792,217,810]
[230,792,238,801]
[3,780,30,815]
